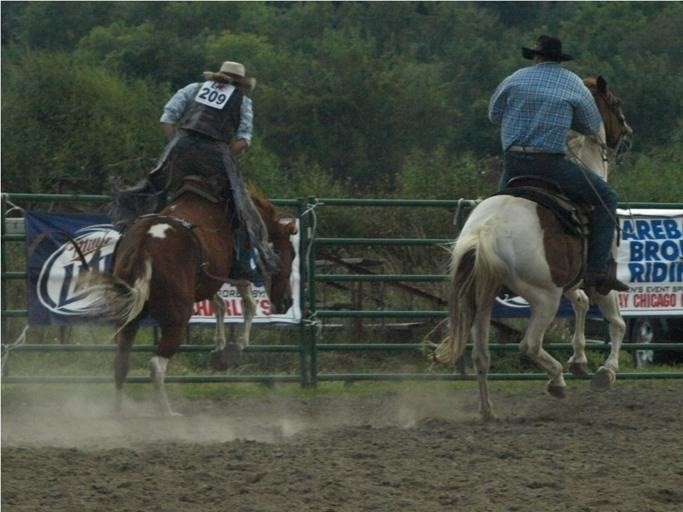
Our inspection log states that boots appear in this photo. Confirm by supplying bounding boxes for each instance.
[229,230,264,281]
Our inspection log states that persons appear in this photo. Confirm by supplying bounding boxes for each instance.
[487,35,630,294]
[159,60,269,285]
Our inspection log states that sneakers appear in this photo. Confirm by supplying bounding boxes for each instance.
[584,272,629,292]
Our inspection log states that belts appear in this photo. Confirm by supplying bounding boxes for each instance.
[508,145,544,153]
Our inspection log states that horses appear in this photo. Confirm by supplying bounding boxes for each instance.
[81,184,299,422]
[421,75,634,425]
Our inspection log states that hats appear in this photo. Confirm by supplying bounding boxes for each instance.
[521,36,575,62]
[203,61,257,91]
